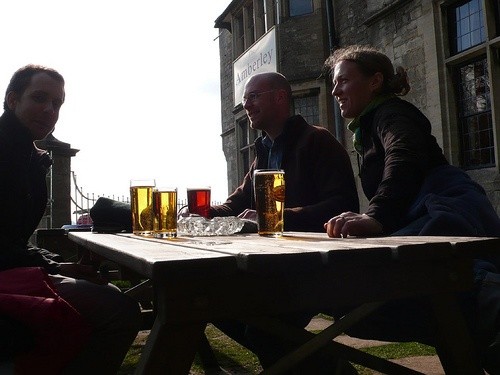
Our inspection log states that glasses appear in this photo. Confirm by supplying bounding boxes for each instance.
[243,90,278,101]
[16,92,61,107]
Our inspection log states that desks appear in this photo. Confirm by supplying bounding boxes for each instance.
[68,231,500,375]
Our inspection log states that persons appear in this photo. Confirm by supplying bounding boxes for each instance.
[0,65,141,375]
[323,45,500,375]
[177,73,360,332]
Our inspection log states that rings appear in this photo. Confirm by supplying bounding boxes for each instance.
[340,216,348,222]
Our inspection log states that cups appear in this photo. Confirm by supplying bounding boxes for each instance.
[152,187,178,238]
[130,179,156,235]
[186,185,211,221]
[254,169,286,238]
